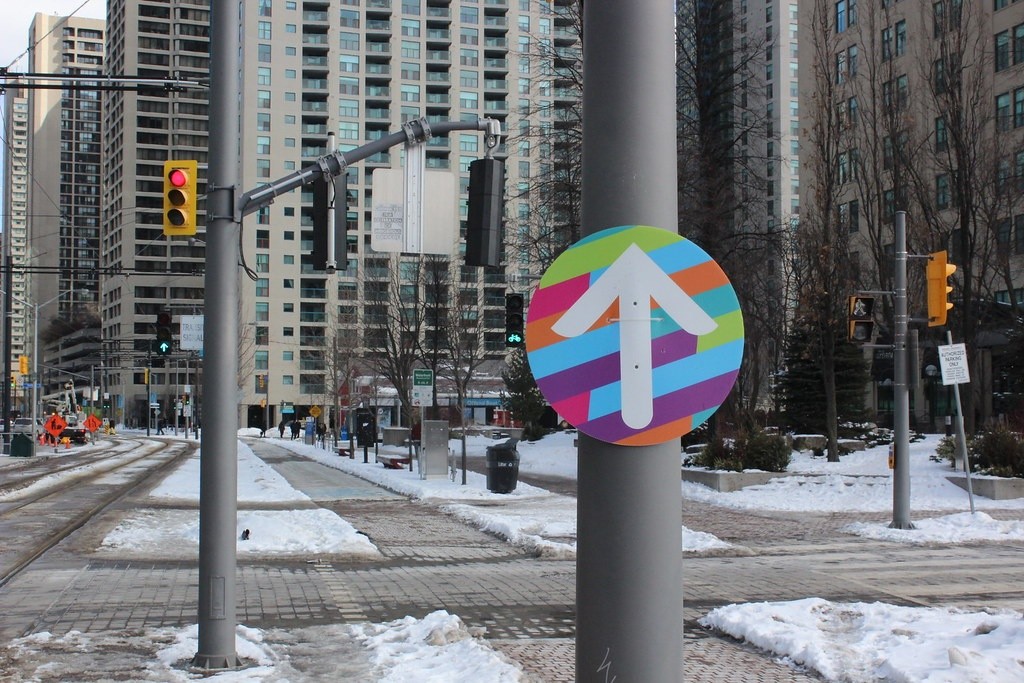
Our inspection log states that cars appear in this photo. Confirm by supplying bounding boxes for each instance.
[56,415,86,445]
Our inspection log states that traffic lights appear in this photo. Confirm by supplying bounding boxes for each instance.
[181,395,186,405]
[173,402,176,411]
[103,400,110,409]
[260,399,266,408]
[162,161,198,236]
[155,310,175,355]
[260,375,264,388]
[186,395,190,405]
[10,376,16,388]
[848,296,878,343]
[888,445,894,469]
[503,291,526,345]
[926,251,957,328]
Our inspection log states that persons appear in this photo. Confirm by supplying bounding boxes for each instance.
[260,420,267,437]
[156,419,164,435]
[110,418,115,435]
[290,420,298,440]
[317,424,324,442]
[279,422,285,438]
[296,419,301,438]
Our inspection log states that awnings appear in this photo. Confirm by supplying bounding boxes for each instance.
[282,408,294,413]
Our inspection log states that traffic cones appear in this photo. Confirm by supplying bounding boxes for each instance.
[65,438,72,448]
[54,437,59,447]
[40,432,46,445]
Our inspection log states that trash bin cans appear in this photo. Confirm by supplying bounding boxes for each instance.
[11,432,34,457]
[486,438,523,494]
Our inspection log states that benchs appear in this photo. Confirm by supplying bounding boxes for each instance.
[333,446,352,456]
[377,454,410,469]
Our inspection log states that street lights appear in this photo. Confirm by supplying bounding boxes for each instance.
[0,289,89,455]
[924,363,938,434]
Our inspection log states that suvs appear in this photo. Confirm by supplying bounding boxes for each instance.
[0,418,45,437]
